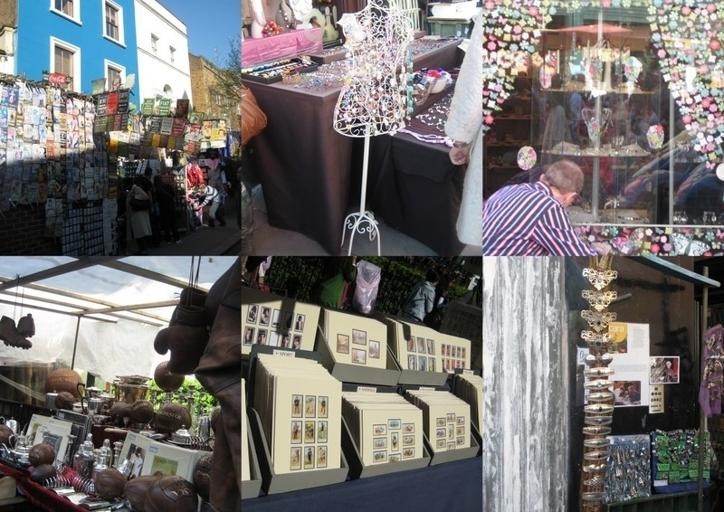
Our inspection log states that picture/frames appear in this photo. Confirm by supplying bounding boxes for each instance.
[20,405,200,500]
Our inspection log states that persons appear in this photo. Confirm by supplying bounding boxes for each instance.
[196,184,226,228]
[240,255,273,292]
[615,383,639,403]
[307,255,360,314]
[280,388,331,469]
[243,302,306,353]
[481,160,596,256]
[126,167,178,254]
[397,268,436,326]
[501,57,670,202]
[432,267,462,329]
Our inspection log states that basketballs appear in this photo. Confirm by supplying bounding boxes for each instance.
[0,424,16,449]
[132,399,153,424]
[124,476,156,511]
[110,401,131,427]
[95,469,126,498]
[155,402,191,433]
[29,443,55,467]
[154,361,185,391]
[145,476,198,512]
[193,454,213,500]
[55,392,75,410]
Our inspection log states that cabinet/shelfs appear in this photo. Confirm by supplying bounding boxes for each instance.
[486,25,535,196]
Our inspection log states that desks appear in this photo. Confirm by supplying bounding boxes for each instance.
[1,444,118,510]
[240,28,462,255]
[358,66,463,255]
